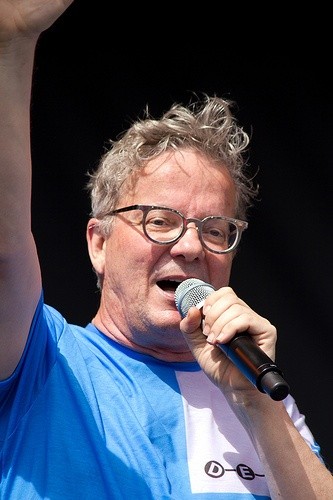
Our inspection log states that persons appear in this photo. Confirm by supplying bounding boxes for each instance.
[0,0,333,500]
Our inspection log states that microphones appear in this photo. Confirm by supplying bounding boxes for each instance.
[175,278,290,401]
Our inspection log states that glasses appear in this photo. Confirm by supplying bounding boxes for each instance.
[106,204,248,254]
